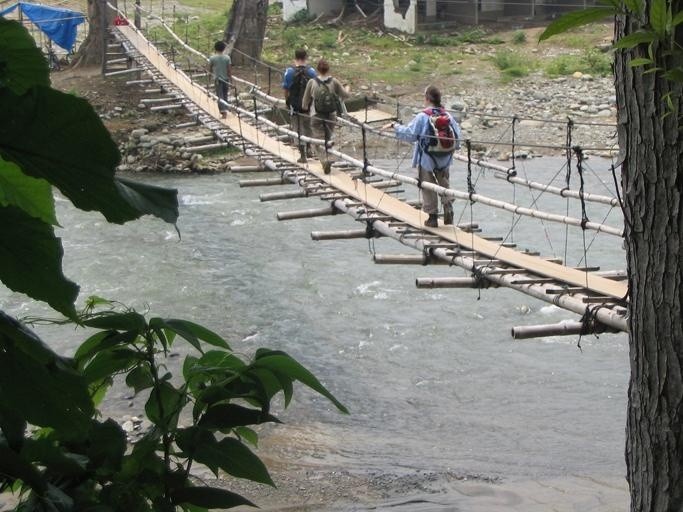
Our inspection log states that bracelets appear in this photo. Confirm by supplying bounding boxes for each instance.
[391,122,395,128]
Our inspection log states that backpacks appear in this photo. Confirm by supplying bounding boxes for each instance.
[314,77,336,113]
[286,64,312,114]
[418,108,456,153]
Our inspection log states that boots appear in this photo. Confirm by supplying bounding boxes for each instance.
[297,145,317,163]
[444,202,453,225]
[424,214,437,227]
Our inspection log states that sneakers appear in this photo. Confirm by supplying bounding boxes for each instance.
[323,161,330,174]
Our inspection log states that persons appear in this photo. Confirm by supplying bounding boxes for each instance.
[114,10,128,26]
[379,85,461,227]
[281,48,314,163]
[205,40,233,119]
[302,59,350,174]
